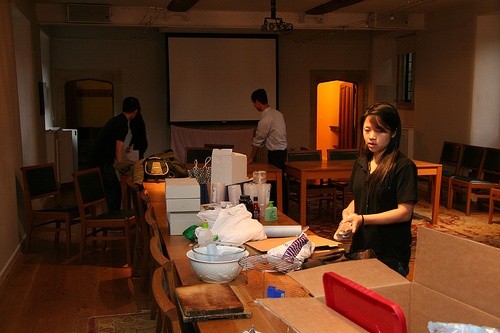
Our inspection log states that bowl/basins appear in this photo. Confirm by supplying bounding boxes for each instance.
[186,246,249,284]
[192,242,245,262]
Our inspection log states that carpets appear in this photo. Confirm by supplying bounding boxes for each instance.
[85,310,155,333]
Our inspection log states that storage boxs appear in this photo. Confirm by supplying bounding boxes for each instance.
[254,230,500,333]
[164,176,201,237]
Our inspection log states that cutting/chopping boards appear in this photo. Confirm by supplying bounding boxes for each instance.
[178,286,252,323]
[176,282,244,317]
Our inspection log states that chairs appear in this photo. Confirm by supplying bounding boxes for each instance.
[139,187,183,333]
[184,143,238,164]
[72,167,138,266]
[435,140,500,225]
[286,149,336,225]
[326,150,363,222]
[20,163,78,255]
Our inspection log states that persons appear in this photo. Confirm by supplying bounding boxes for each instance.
[247,89,289,216]
[96,96,141,211]
[125,107,148,160]
[339,101,418,279]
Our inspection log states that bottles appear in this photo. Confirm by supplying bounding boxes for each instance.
[252,197,260,222]
[198,222,213,247]
[259,204,265,217]
[239,195,253,219]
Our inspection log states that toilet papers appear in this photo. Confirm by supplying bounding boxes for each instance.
[264,225,302,237]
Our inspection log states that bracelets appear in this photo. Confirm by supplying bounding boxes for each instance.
[360,214,364,226]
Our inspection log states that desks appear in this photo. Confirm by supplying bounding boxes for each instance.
[143,180,346,333]
[285,159,443,227]
[180,161,283,213]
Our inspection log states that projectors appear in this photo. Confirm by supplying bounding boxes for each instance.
[261,23,293,31]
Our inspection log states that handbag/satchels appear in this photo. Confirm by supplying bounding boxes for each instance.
[144,150,188,182]
[267,232,315,270]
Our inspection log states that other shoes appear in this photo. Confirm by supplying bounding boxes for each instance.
[101,226,126,232]
